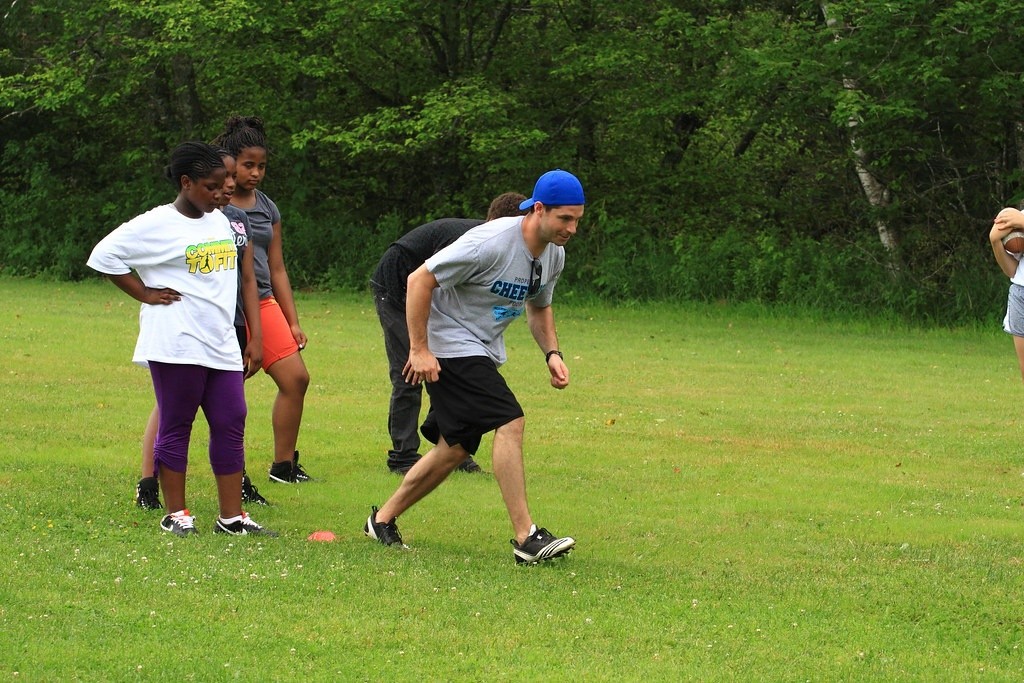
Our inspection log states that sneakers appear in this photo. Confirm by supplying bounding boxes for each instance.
[363,506,412,551]
[269,450,317,484]
[158,508,199,537]
[136,476,163,513]
[509,523,576,564]
[456,456,490,475]
[212,512,279,539]
[240,474,270,507]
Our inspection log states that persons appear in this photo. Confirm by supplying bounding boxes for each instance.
[213,114,311,510]
[85,140,280,540]
[368,191,532,481]
[989,207,1024,386]
[363,168,584,570]
[134,141,271,515]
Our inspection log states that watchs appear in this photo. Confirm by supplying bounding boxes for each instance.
[545,350,563,363]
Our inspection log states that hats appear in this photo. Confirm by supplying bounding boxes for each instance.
[519,169,585,211]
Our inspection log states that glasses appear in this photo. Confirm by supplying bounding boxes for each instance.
[529,257,542,295]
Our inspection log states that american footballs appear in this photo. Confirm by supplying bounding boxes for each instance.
[997,207,1024,253]
[308,531,335,542]
